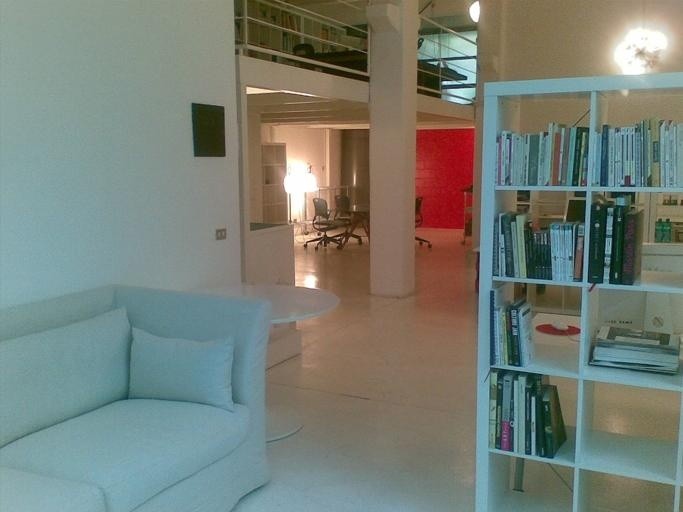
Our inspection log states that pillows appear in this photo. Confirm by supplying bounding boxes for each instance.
[127,325,236,414]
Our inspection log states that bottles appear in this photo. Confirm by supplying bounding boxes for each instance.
[655,218,672,243]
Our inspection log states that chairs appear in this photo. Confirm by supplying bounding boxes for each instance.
[304,195,432,251]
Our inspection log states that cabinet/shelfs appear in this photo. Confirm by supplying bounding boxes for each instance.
[262,142,289,225]
[473,70,682,512]
[235,0,371,69]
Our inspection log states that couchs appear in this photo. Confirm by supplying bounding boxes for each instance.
[0,285,270,511]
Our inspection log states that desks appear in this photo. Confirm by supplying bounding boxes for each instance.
[267,285,339,446]
[295,51,468,99]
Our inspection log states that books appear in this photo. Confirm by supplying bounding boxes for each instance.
[589,326,680,375]
[488,368,567,458]
[493,197,645,285]
[490,282,532,367]
[496,118,683,188]
